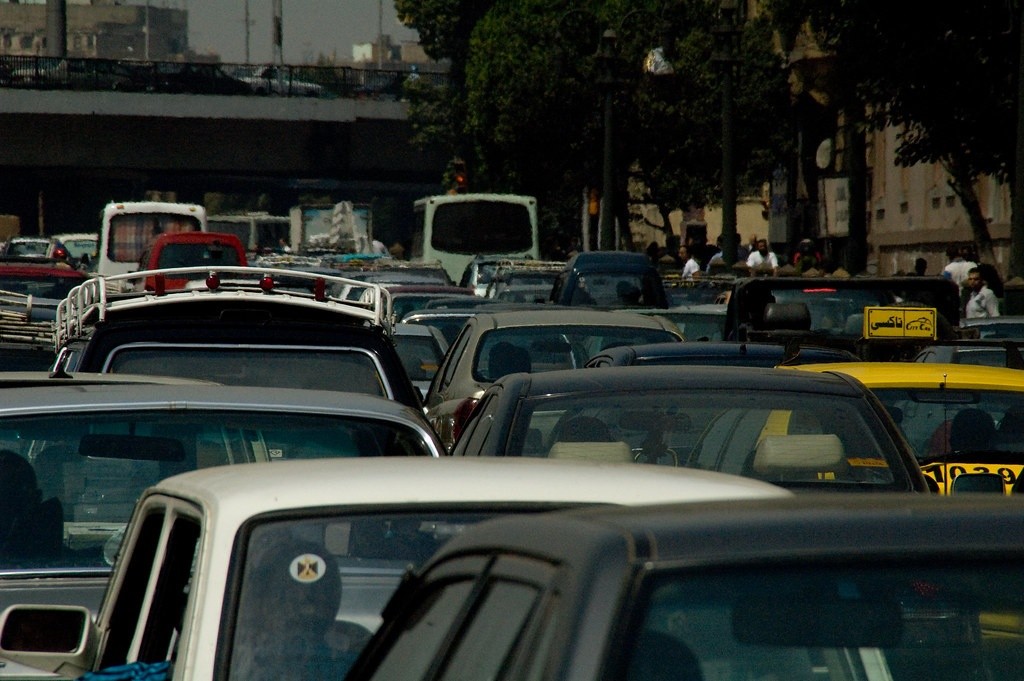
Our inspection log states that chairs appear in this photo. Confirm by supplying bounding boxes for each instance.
[547,441,634,465]
[754,433,846,472]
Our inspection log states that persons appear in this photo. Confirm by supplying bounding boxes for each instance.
[253,244,260,250]
[487,342,512,378]
[915,258,927,276]
[965,269,999,317]
[746,240,779,273]
[279,238,290,251]
[647,233,745,278]
[942,248,976,298]
[794,239,821,268]
[409,66,420,81]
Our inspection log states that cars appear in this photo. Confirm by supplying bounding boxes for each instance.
[0,165,1024,681]
[0,43,326,101]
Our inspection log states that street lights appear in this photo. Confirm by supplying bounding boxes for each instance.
[552,4,673,252]
